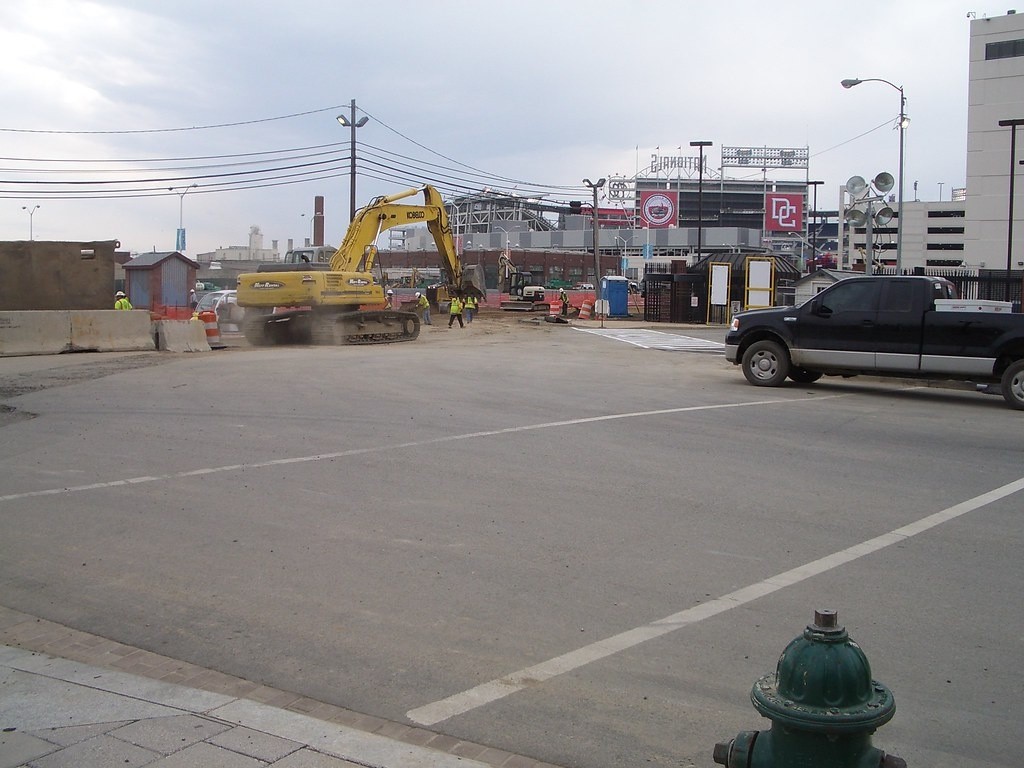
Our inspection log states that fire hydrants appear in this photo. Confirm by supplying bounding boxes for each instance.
[714,608,907,768]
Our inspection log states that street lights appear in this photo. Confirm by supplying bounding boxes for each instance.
[168,182,197,253]
[495,225,521,277]
[583,179,607,299]
[843,78,911,276]
[787,232,803,276]
[615,236,638,276]
[299,211,321,245]
[22,205,41,241]
[630,214,666,259]
[998,118,1024,302]
[807,182,825,273]
[690,142,713,261]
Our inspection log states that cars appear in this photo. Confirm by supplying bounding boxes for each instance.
[629,278,670,292]
[581,284,595,289]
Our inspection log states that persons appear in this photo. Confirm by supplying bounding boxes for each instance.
[415,292,433,325]
[447,293,478,330]
[190,289,198,309]
[383,289,393,310]
[558,287,570,317]
[113,291,133,311]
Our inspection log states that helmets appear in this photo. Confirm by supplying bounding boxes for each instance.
[387,290,393,294]
[559,287,563,292]
[114,291,126,296]
[415,291,420,298]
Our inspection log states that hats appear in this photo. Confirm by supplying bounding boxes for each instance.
[189,289,195,292]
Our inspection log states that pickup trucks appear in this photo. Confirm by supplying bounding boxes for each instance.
[724,276,1024,410]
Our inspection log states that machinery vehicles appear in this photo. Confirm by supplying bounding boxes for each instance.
[497,253,550,311]
[393,268,425,288]
[257,246,340,271]
[237,184,488,346]
